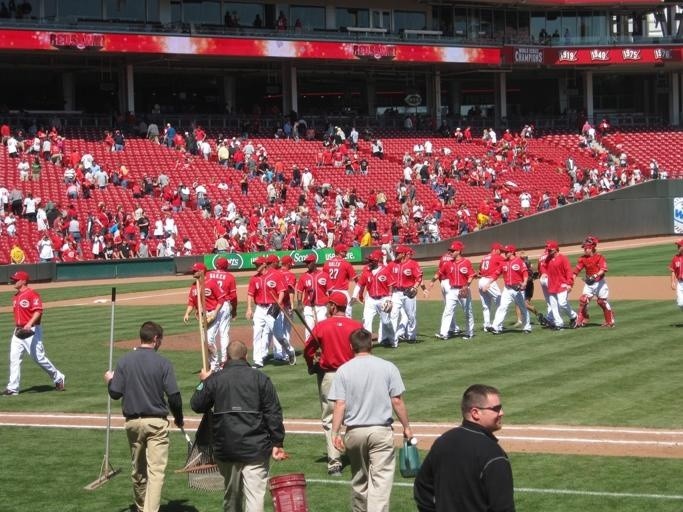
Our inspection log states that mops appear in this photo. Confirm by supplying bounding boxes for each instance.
[84,288,122,489]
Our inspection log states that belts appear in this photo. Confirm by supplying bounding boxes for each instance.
[126,415,167,422]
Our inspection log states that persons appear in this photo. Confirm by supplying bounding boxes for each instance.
[0,271,65,395]
[305,291,413,512]
[190,339,285,512]
[0,0,662,265]
[429,236,615,340]
[105,322,183,512]
[669,239,682,309]
[414,384,515,512]
[182,244,428,372]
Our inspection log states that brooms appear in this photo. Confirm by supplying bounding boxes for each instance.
[132,345,206,469]
[187,267,227,493]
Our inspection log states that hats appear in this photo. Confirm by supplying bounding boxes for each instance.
[491,243,516,252]
[334,244,349,253]
[322,292,348,307]
[545,240,559,251]
[365,250,387,262]
[214,258,231,268]
[301,255,316,263]
[448,240,464,250]
[187,262,208,273]
[675,240,683,246]
[391,245,416,255]
[9,271,29,280]
[581,236,599,250]
[252,254,295,265]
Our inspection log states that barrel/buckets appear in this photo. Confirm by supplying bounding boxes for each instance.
[397,433,426,476]
[268,451,310,512]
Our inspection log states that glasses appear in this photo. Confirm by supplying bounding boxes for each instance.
[478,405,502,413]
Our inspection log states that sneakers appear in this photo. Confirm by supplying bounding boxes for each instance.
[484,325,501,334]
[56,374,65,390]
[274,352,289,361]
[289,349,297,366]
[253,364,263,368]
[378,339,398,348]
[0,388,19,395]
[511,314,578,334]
[327,464,342,477]
[398,332,415,342]
[435,325,473,340]
